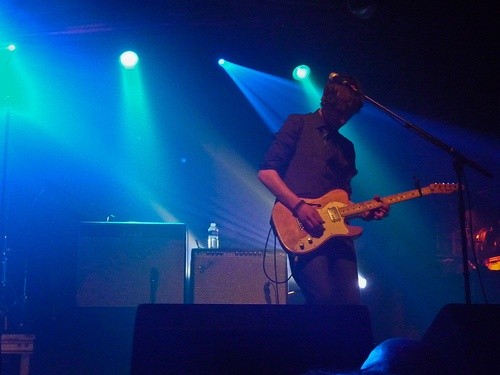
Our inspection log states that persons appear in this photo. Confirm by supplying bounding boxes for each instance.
[258,75,390,305]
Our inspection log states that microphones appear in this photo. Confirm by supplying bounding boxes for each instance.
[329,72,362,93]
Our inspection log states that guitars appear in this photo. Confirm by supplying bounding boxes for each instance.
[271,182,465,260]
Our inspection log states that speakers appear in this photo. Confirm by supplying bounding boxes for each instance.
[73,221,186,307]
[419,303,500,375]
[191,248,289,304]
[129,303,375,375]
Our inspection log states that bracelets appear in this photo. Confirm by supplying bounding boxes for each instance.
[293,200,305,216]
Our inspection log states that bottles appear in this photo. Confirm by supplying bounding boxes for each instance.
[207,222,220,249]
